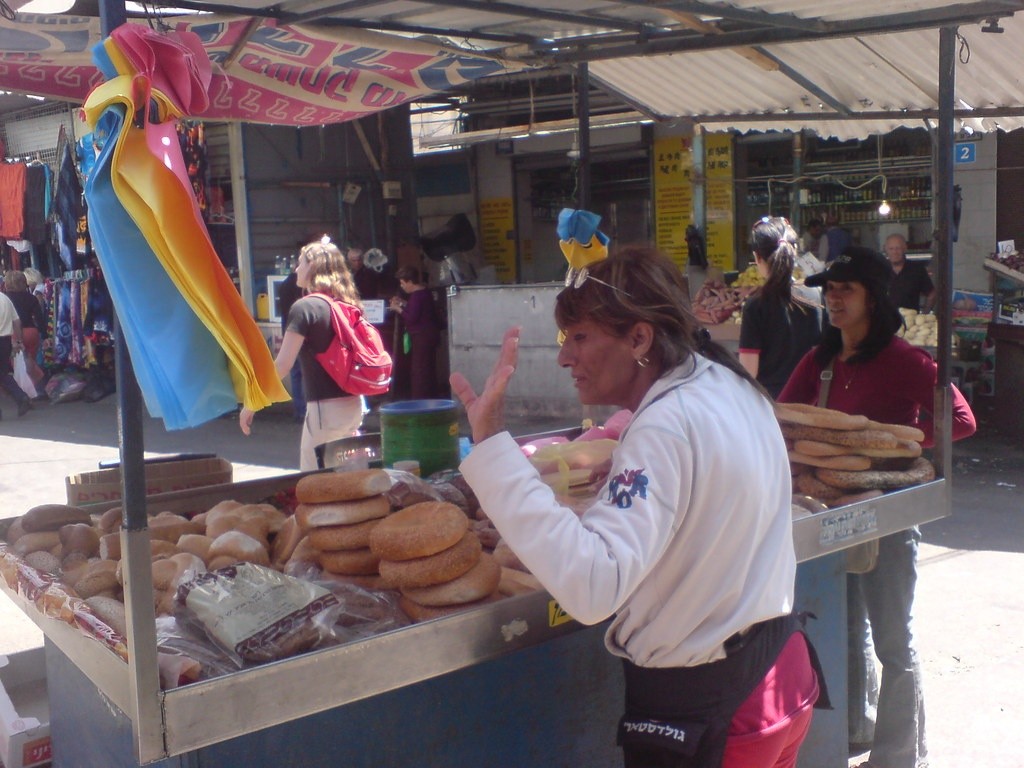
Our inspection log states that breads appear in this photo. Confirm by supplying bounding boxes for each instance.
[773,403,934,520]
[3,439,622,688]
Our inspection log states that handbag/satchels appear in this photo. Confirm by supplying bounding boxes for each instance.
[846,538,879,573]
[404,332,410,354]
[528,439,619,520]
[13,349,38,399]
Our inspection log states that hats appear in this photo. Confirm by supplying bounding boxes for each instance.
[804,248,892,287]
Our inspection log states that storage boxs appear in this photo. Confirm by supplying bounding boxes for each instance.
[65,453,233,507]
[0,646,53,768]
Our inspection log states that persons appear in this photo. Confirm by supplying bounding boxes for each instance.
[347,247,377,299]
[24,268,46,314]
[2,270,48,409]
[240,240,365,471]
[447,247,833,768]
[885,233,937,314]
[278,239,314,423]
[0,292,34,420]
[774,246,977,768]
[738,216,827,401]
[385,265,441,399]
[801,212,853,263]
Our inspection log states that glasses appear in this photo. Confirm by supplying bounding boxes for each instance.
[565,266,644,304]
[752,217,790,242]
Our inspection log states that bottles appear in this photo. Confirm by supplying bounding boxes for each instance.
[393,461,420,480]
[801,177,931,225]
[272,255,296,275]
[257,292,269,320]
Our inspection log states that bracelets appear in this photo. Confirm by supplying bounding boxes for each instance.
[16,340,23,343]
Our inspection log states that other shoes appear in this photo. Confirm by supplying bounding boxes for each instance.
[19,401,32,416]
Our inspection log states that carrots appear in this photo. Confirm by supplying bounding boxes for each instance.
[691,278,759,323]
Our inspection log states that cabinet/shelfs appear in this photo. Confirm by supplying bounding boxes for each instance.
[775,196,932,226]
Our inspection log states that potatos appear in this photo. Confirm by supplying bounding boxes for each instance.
[895,308,958,347]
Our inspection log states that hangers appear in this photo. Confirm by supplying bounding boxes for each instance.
[50,269,94,283]
[27,152,43,167]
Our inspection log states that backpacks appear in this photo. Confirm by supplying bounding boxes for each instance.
[304,293,391,395]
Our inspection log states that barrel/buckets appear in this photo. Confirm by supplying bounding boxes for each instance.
[380,399,460,473]
